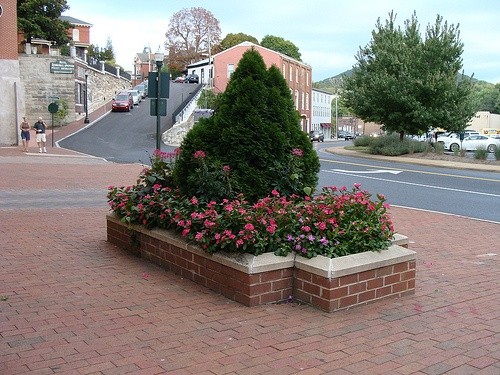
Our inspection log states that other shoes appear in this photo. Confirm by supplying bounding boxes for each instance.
[26,149,28,152]
[44,149,46,153]
[22,149,24,152]
[39,150,42,152]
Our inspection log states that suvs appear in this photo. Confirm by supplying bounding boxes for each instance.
[309,130,324,142]
[187,74,199,83]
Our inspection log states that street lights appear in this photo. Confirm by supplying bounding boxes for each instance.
[335,95,342,140]
[83,71,89,123]
[154,44,165,150]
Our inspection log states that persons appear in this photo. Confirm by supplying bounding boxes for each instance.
[20,116,30,152]
[33,117,48,153]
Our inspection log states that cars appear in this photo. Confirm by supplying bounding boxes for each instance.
[412,129,500,153]
[111,79,148,112]
[338,132,356,140]
[174,77,187,82]
